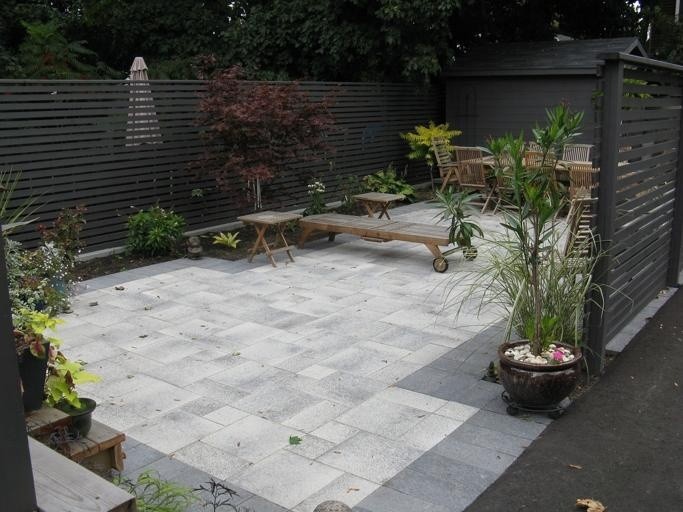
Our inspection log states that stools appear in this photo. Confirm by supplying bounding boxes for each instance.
[354,194,404,245]
[236,211,303,268]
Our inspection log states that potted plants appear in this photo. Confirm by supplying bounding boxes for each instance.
[474,105,603,407]
[48,354,102,436]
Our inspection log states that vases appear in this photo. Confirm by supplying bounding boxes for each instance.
[16,341,55,412]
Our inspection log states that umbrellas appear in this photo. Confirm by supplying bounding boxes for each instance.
[123,57,162,148]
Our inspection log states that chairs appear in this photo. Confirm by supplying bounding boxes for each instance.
[544,196,598,308]
[430,135,601,211]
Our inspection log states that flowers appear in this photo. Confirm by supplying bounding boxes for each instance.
[2,245,76,357]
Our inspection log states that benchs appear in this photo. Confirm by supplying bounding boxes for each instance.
[295,212,477,273]
